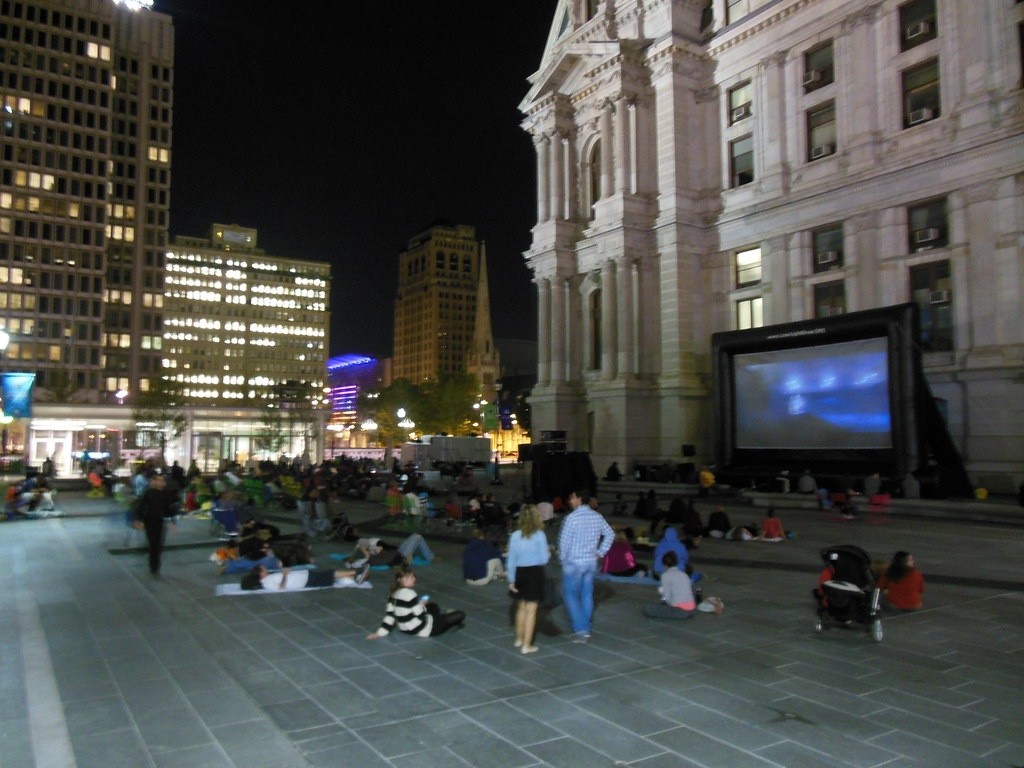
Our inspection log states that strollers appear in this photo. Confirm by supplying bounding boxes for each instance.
[813,544,889,642]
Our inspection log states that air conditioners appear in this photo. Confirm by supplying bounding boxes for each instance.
[819,252,837,264]
[917,228,939,243]
[802,71,820,85]
[735,105,748,118]
[910,108,933,125]
[907,21,929,40]
[929,289,951,304]
[812,145,832,159]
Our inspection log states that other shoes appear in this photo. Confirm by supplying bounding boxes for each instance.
[498,573,506,583]
[570,630,591,644]
[522,645,539,655]
[432,554,444,562]
[514,638,523,648]
[355,563,370,584]
[701,575,717,581]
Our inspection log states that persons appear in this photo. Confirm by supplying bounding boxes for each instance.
[365,567,466,640]
[4,450,126,521]
[121,445,926,626]
[506,503,552,654]
[556,489,615,642]
